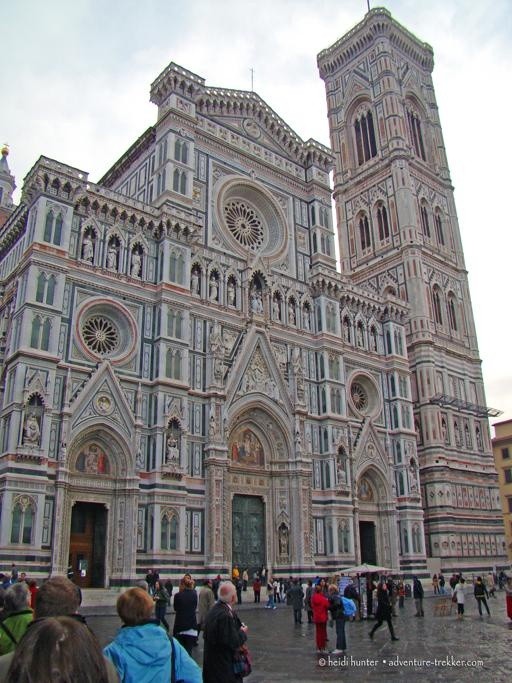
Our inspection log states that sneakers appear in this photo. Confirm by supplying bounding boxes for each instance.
[391,636,400,641]
[317,649,329,654]
[368,632,374,640]
[331,649,344,654]
[266,605,277,609]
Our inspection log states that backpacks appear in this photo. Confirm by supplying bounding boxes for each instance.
[336,594,357,617]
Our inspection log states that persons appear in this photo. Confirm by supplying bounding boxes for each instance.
[356,327,363,347]
[190,269,310,330]
[343,321,349,343]
[231,430,265,467]
[131,249,142,277]
[83,234,94,261]
[334,455,347,484]
[369,331,376,350]
[107,243,117,270]
[166,431,180,461]
[0,562,511,682]
[24,410,40,448]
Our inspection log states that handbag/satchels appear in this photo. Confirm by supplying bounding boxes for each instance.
[452,591,457,604]
[233,643,253,677]
